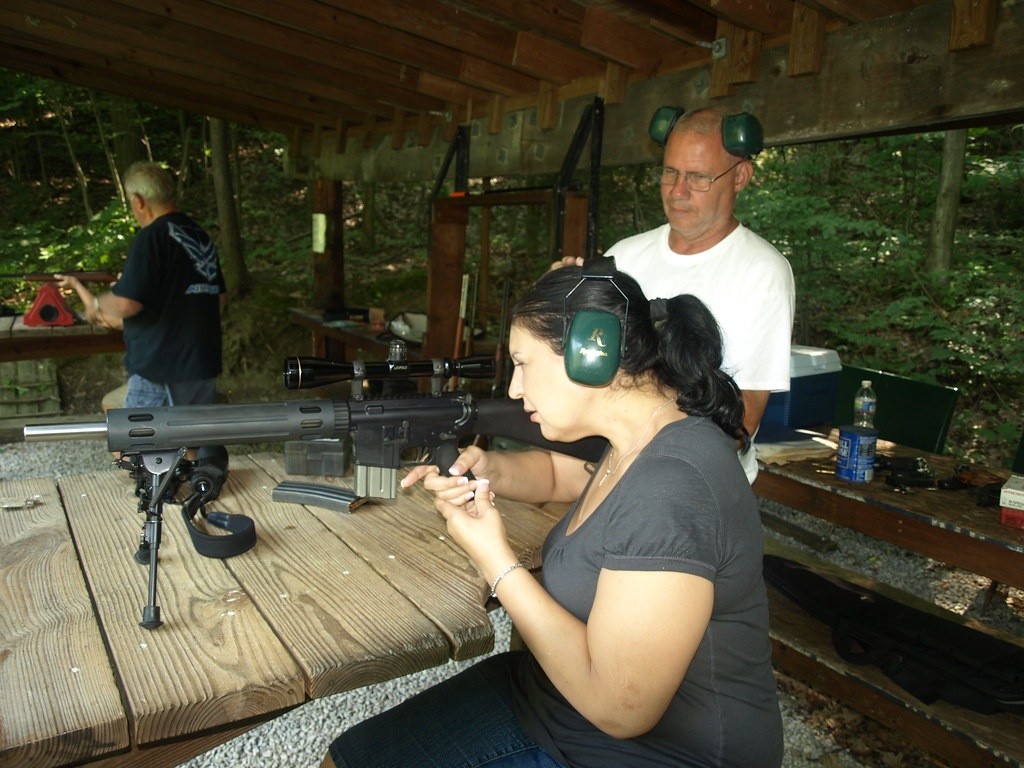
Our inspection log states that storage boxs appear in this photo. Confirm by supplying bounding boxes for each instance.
[1001,507,1024,529]
[999,474,1024,510]
[752,345,842,443]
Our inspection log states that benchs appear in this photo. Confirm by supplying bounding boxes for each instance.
[761,532,1024,768]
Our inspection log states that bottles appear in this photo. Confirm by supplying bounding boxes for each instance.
[853,380,877,429]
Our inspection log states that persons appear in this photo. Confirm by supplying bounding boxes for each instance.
[316,253,785,768]
[55,160,230,471]
[549,105,798,485]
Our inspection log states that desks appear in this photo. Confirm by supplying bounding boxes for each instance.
[738,425,1024,767]
[0,316,127,362]
[286,303,505,396]
[0,469,558,768]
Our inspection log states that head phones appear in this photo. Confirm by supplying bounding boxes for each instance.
[561,256,629,389]
[648,106,765,160]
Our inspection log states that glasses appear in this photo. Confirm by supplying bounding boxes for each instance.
[655,158,743,192]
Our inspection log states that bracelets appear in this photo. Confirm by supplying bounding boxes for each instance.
[91,295,102,314]
[490,563,525,597]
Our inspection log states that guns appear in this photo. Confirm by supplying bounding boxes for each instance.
[22,354,628,633]
[492,281,511,397]
[0,268,120,288]
[444,274,469,392]
[464,271,484,394]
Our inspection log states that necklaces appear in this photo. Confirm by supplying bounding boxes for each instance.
[595,396,676,487]
